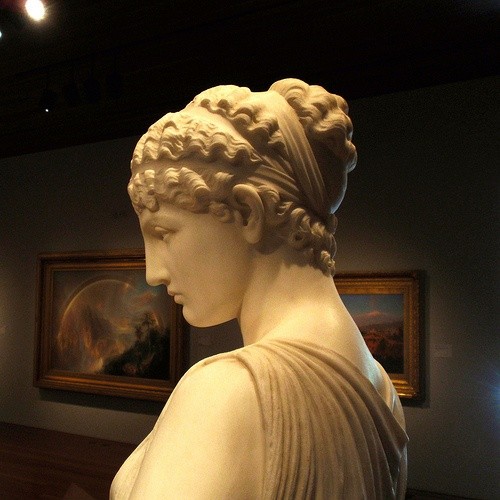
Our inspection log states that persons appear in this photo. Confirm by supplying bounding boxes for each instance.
[104,79,409,500]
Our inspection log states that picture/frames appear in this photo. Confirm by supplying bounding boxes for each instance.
[333,268,424,405]
[29,249,188,405]
